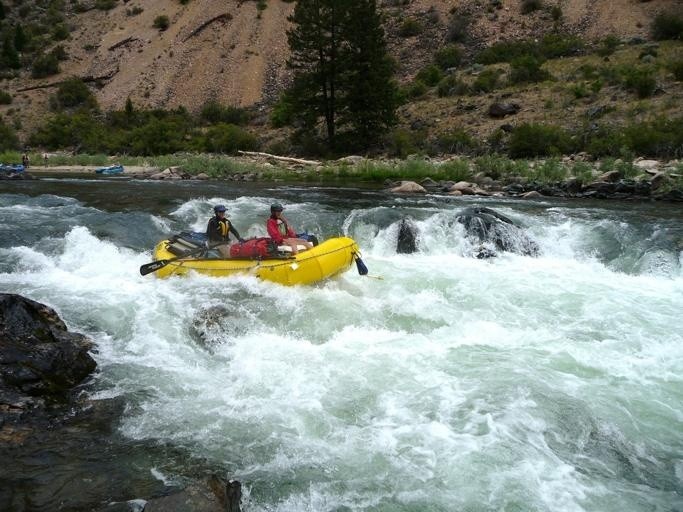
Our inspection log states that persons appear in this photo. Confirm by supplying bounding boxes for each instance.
[22,154,27,169]
[266,204,313,256]
[43,154,48,168]
[26,151,30,169]
[206,205,245,260]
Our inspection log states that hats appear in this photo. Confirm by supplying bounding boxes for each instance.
[214,205,229,211]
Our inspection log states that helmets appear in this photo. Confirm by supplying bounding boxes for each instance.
[270,203,282,210]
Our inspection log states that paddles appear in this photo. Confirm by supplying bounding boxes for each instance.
[139,243,228,276]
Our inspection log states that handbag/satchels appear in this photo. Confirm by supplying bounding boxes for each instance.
[230,237,276,259]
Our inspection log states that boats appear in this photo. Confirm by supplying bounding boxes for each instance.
[95,166,123,174]
[152,237,359,288]
[0,163,24,172]
